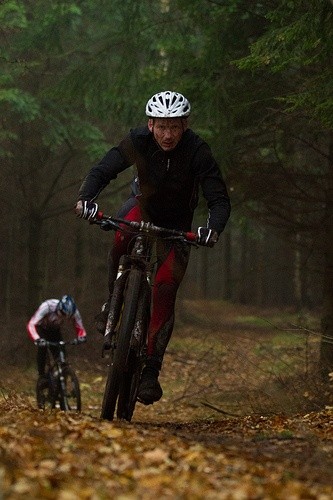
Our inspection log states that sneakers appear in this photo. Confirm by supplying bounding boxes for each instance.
[138,367,162,405]
[95,303,110,334]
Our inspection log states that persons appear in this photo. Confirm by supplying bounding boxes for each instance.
[76,90,232,402]
[27,294,86,411]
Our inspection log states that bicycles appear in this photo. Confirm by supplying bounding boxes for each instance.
[72,203,219,423]
[34,338,87,412]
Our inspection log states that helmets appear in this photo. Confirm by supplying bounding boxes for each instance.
[57,295,77,319]
[145,90,191,118]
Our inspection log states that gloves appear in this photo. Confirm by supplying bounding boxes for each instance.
[197,226,218,249]
[76,200,99,221]
